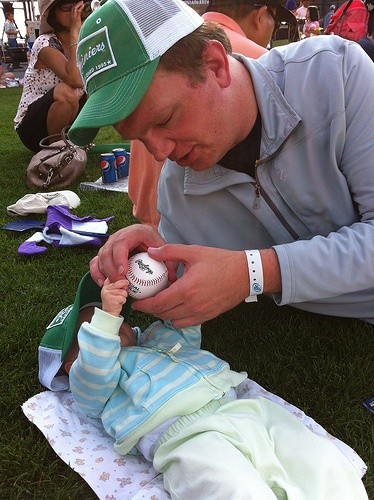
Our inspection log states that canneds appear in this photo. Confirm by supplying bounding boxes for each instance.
[100,152,119,183]
[112,148,128,177]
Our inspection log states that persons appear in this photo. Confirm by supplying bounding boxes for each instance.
[67,0,374,330]
[13,0,89,154]
[264,0,374,51]
[24,18,34,52]
[5,12,24,69]
[38,270,370,500]
[128,0,298,230]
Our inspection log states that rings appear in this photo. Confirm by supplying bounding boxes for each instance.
[73,10,77,12]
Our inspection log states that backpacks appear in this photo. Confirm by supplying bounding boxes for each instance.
[324,0,368,41]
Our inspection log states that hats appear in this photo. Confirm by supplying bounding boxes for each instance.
[67,0,204,147]
[38,271,131,392]
[271,0,298,27]
[38,0,91,35]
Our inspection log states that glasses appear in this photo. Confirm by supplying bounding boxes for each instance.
[253,3,282,35]
[56,3,77,12]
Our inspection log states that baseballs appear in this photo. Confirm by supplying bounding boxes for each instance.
[125,254,168,299]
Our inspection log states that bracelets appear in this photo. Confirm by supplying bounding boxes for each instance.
[244,249,263,302]
[68,42,78,49]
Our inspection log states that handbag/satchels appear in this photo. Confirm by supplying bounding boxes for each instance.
[25,125,88,191]
